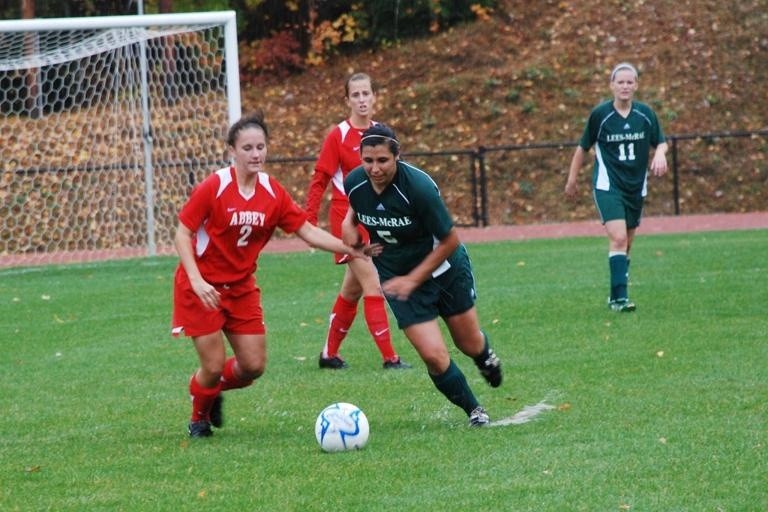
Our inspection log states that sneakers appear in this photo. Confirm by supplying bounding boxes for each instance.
[468,406,490,427]
[319,352,348,369]
[188,421,214,439]
[607,296,636,313]
[209,395,223,428]
[383,357,412,369]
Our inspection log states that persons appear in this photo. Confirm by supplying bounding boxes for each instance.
[565,62,670,313]
[305,67,413,373]
[173,116,385,439]
[341,124,503,428]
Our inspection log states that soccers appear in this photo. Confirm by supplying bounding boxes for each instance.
[315,402,369,453]
